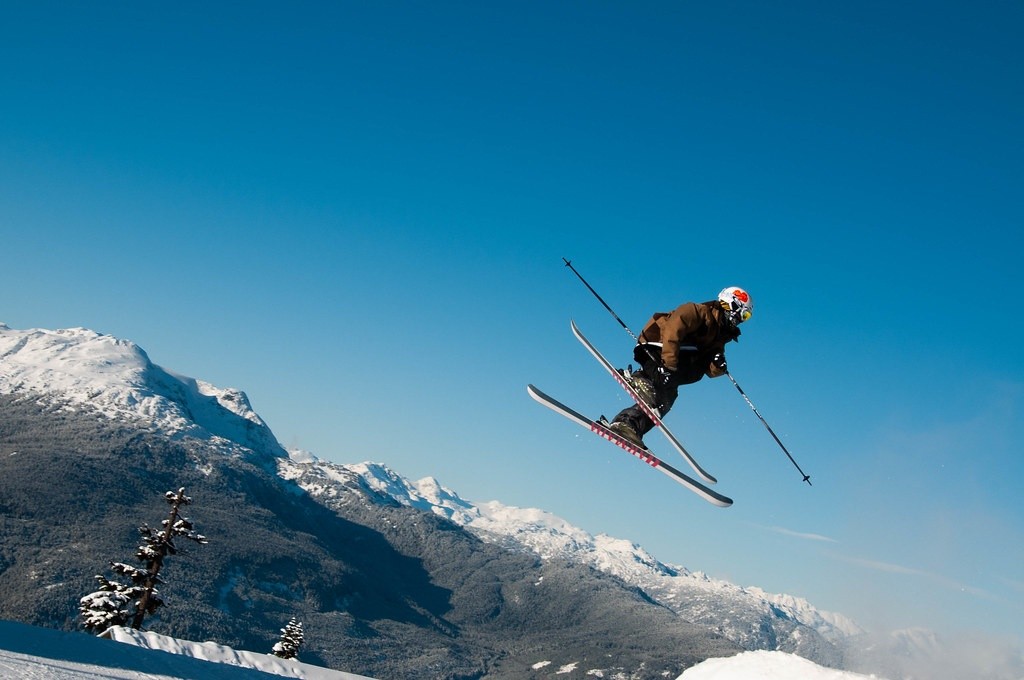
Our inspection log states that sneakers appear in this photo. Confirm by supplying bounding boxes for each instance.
[615,364,663,421]
[596,415,656,458]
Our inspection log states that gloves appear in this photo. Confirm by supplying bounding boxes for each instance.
[705,352,728,379]
[657,363,685,388]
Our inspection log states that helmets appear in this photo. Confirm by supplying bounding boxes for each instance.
[718,286,754,327]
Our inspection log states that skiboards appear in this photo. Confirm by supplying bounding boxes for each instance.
[524,317,734,508]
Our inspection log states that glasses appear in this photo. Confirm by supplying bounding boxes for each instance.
[728,294,752,321]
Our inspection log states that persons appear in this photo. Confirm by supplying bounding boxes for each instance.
[609,286,754,450]
